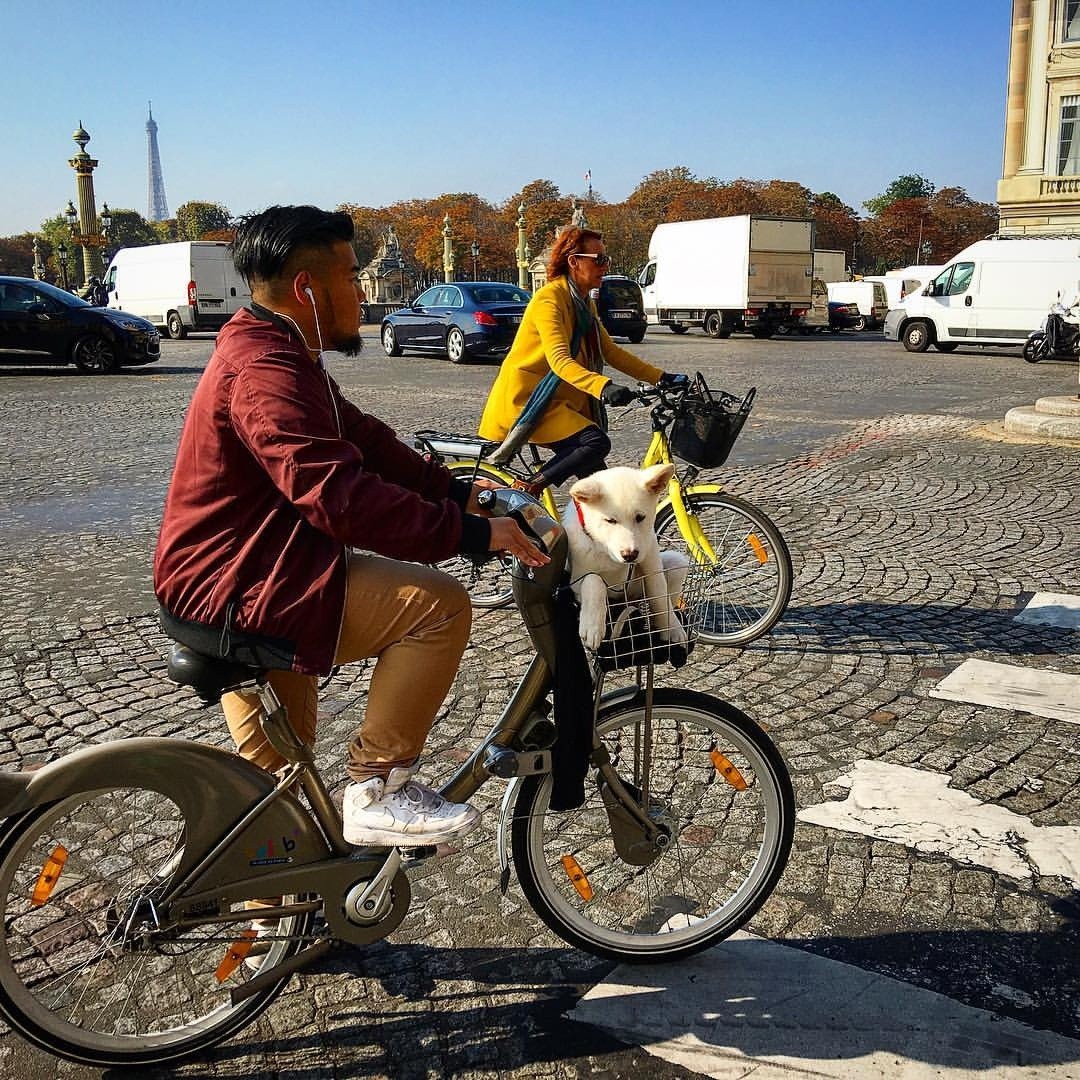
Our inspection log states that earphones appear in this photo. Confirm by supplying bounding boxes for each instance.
[305,288,315,305]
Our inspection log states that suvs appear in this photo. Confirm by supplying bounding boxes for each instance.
[590,276,647,344]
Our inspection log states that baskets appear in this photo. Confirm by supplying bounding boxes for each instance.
[598,564,706,669]
[668,390,752,468]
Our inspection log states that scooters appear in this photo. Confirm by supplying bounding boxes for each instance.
[1019,291,1080,363]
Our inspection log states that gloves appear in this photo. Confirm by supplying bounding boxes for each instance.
[659,373,689,388]
[602,383,632,407]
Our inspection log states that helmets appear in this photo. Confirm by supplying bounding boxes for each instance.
[89,276,96,283]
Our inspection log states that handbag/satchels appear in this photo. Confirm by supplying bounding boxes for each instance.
[680,370,756,468]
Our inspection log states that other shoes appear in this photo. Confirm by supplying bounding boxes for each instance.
[514,480,543,499]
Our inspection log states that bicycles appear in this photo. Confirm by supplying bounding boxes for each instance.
[412,374,797,649]
[3,475,799,1068]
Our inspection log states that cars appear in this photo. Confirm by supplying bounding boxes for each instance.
[0,275,163,373]
[380,281,534,364]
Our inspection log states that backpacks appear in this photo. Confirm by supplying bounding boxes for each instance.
[89,285,109,306]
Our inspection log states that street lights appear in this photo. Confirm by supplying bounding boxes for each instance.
[100,246,111,273]
[56,240,68,291]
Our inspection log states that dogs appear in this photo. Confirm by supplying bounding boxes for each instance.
[561,461,689,651]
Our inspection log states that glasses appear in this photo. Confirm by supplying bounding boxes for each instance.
[573,253,612,267]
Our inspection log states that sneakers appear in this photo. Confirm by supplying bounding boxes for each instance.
[243,910,326,972]
[342,763,482,847]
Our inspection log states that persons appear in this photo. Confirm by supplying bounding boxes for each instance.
[80,276,109,307]
[154,206,551,973]
[2,285,36,312]
[477,227,681,500]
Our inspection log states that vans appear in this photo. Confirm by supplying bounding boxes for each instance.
[776,248,945,337]
[882,239,1080,353]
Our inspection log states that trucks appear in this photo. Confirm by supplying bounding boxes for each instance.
[104,240,253,337]
[639,213,817,339]
